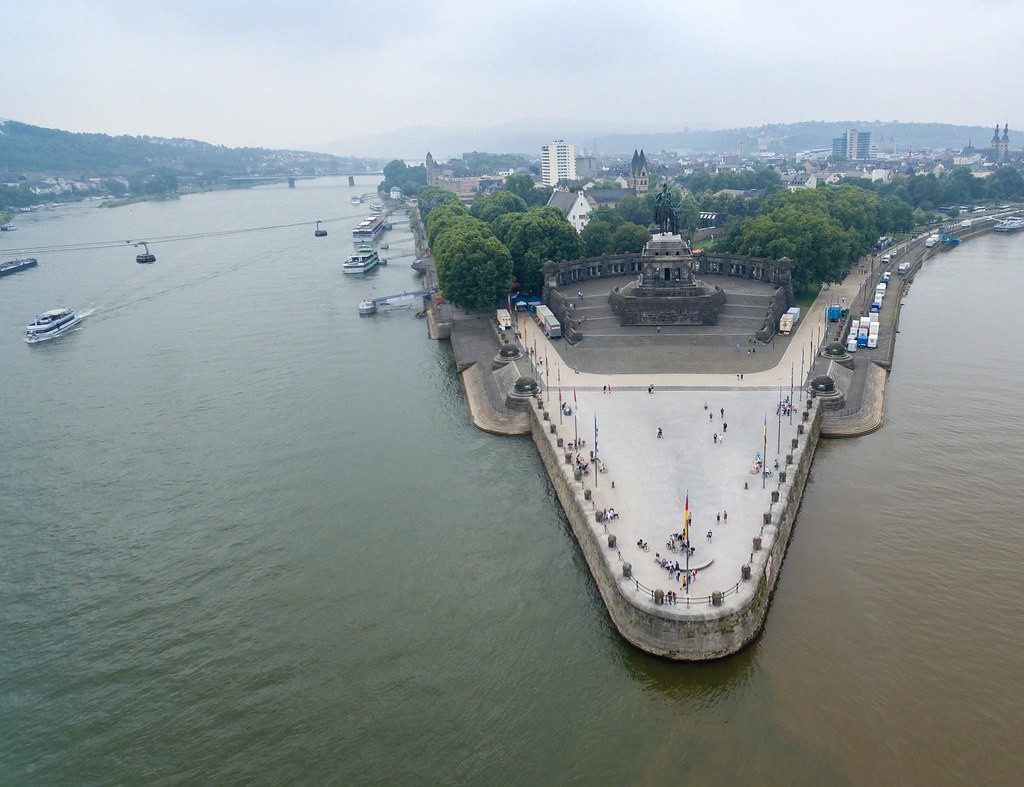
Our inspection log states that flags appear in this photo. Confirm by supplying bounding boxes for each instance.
[683,496,688,545]
[544,349,549,377]
[508,293,512,312]
[802,293,833,363]
[573,389,577,416]
[594,416,599,452]
[791,368,793,396]
[558,366,563,398]
[778,391,782,421]
[763,418,768,454]
[523,319,527,341]
[514,305,518,323]
[532,336,536,356]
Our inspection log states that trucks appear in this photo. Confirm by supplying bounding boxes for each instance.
[536,305,562,339]
[882,254,890,263]
[496,308,512,328]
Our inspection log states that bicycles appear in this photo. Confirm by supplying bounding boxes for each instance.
[640,542,650,552]
[679,545,694,558]
[666,541,678,554]
[762,471,773,479]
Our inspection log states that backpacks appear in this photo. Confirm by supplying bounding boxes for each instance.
[678,572,680,576]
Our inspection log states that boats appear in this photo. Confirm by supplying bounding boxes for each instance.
[350,196,390,243]
[994,215,1024,233]
[0,258,38,275]
[342,243,379,273]
[358,298,377,316]
[0,222,17,231]
[22,306,83,344]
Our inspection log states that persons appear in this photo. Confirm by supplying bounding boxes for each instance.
[654,184,681,236]
[500,290,796,604]
[842,297,845,303]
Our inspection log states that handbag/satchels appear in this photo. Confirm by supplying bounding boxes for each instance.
[683,584,686,587]
